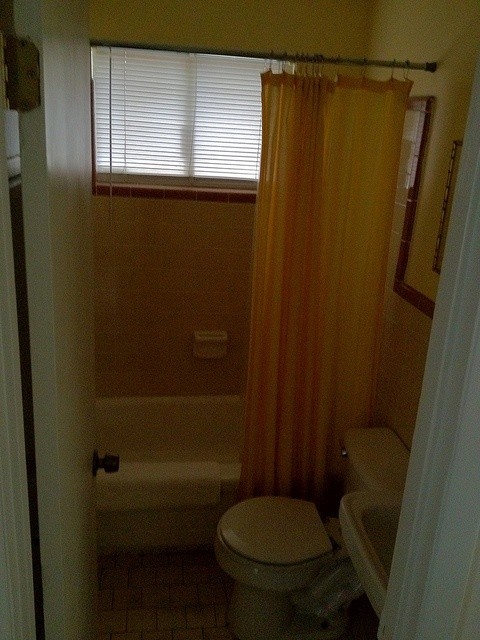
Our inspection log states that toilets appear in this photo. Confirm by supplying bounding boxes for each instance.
[213,427,412,640]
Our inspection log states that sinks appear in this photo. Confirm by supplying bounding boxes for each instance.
[338,489,403,620]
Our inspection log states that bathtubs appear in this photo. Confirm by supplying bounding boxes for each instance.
[95,395,244,547]
